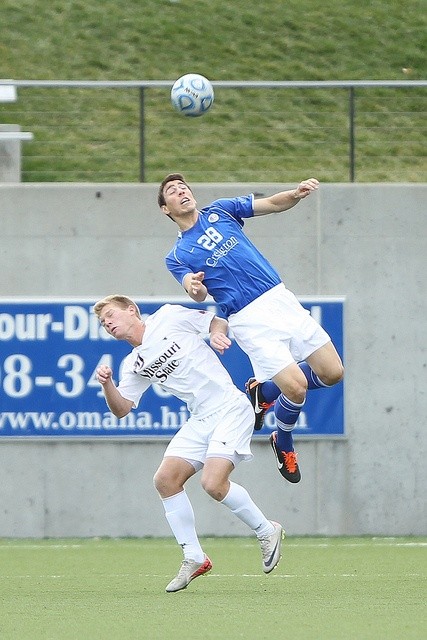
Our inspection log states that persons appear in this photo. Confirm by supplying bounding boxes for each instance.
[91,294,286,593]
[157,176,346,484]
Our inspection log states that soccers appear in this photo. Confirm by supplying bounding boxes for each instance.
[169,73,213,117]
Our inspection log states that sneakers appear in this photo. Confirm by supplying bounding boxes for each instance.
[269,431,301,484]
[166,554,212,592]
[257,521,286,574]
[245,377,276,430]
